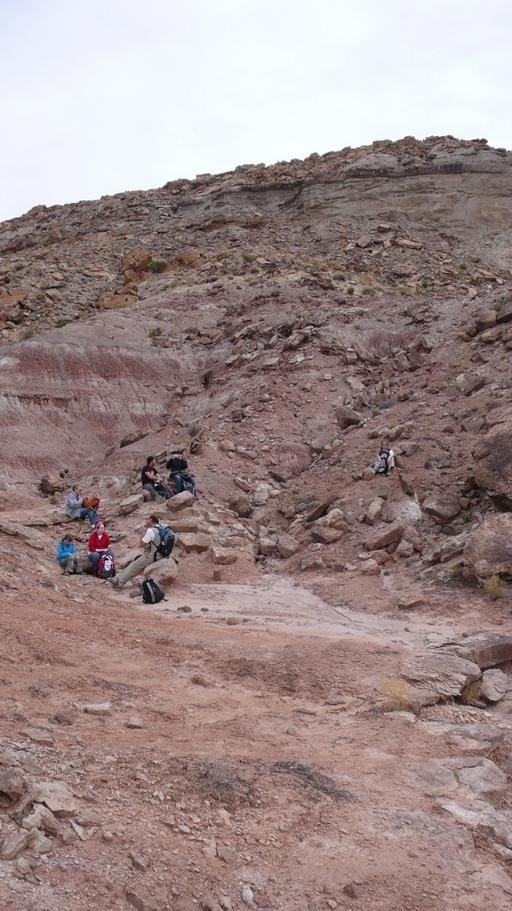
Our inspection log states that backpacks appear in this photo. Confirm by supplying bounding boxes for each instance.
[153,481,173,500]
[376,448,390,474]
[150,526,176,559]
[140,576,165,604]
[96,552,115,580]
[82,495,101,512]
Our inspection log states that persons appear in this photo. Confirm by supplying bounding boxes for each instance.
[64,482,100,529]
[106,514,167,588]
[141,454,172,503]
[165,451,199,500]
[87,519,116,579]
[352,438,396,483]
[56,533,83,576]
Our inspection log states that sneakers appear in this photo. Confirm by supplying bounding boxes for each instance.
[63,572,70,577]
[386,468,393,476]
[107,577,122,589]
[90,524,95,529]
[75,571,87,576]
[352,473,361,482]
